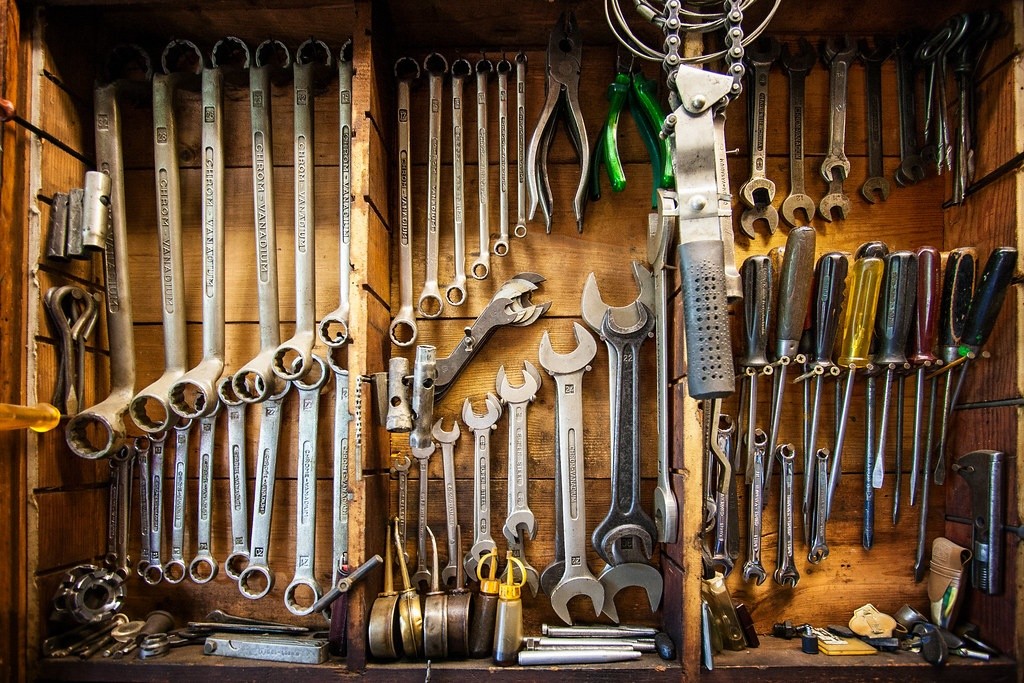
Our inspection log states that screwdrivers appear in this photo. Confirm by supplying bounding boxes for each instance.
[737,226,1016,584]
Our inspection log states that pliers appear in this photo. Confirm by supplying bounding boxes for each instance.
[593,34,675,209]
[527,8,594,236]
